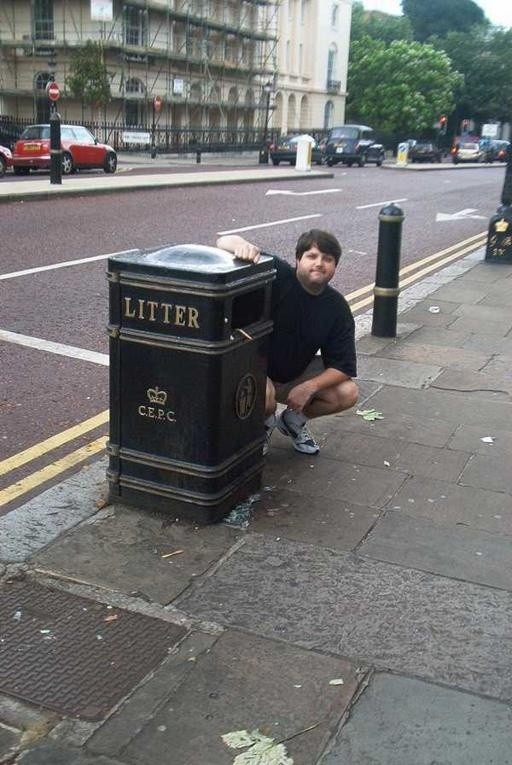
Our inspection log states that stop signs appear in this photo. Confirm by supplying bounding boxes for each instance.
[46,81,60,101]
[154,96,161,110]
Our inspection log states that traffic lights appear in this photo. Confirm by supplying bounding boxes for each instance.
[439,115,448,134]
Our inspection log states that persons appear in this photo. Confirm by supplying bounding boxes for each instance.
[215,229,360,459]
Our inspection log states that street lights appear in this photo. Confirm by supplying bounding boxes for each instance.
[262,79,272,145]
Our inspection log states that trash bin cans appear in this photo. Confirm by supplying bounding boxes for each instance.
[105,242,277,527]
[397,143,410,167]
[295,139,312,171]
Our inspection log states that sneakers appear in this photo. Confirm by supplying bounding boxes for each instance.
[276,406,320,455]
[261,413,279,456]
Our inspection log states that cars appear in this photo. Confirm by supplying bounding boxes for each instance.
[269,124,385,167]
[0,123,118,179]
[394,139,511,163]
